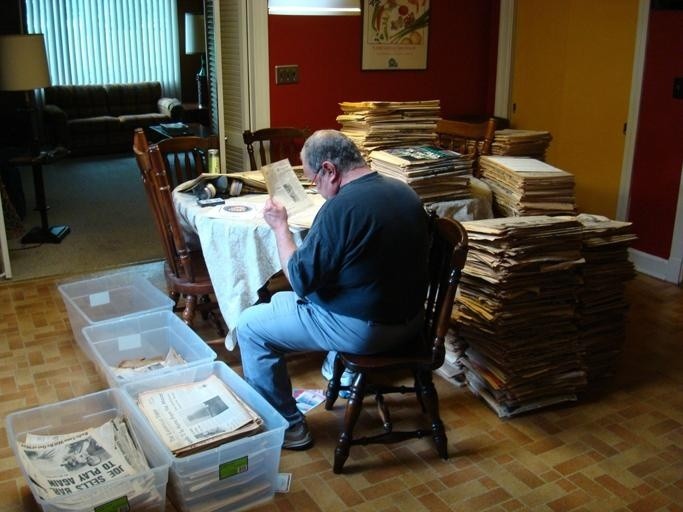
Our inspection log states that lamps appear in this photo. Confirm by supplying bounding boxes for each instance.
[0,34,51,159]
[183,12,207,110]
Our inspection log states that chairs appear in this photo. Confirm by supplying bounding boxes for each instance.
[324,212,469,475]
[432,116,495,181]
[124,125,311,339]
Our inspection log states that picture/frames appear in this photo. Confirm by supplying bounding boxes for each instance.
[359,0,429,71]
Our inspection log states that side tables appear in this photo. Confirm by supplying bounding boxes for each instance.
[0,148,70,246]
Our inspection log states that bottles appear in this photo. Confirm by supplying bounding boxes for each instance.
[208,148,220,175]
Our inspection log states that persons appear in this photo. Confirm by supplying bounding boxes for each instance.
[230,129,438,453]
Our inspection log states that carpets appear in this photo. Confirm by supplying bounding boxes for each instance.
[0,154,166,284]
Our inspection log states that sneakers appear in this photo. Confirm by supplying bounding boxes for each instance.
[282,409,313,450]
[321,360,357,399]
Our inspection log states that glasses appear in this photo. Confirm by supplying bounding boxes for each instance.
[309,165,322,192]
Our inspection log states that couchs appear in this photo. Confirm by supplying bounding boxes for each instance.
[39,83,183,166]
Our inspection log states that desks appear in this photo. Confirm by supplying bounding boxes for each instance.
[169,171,491,337]
